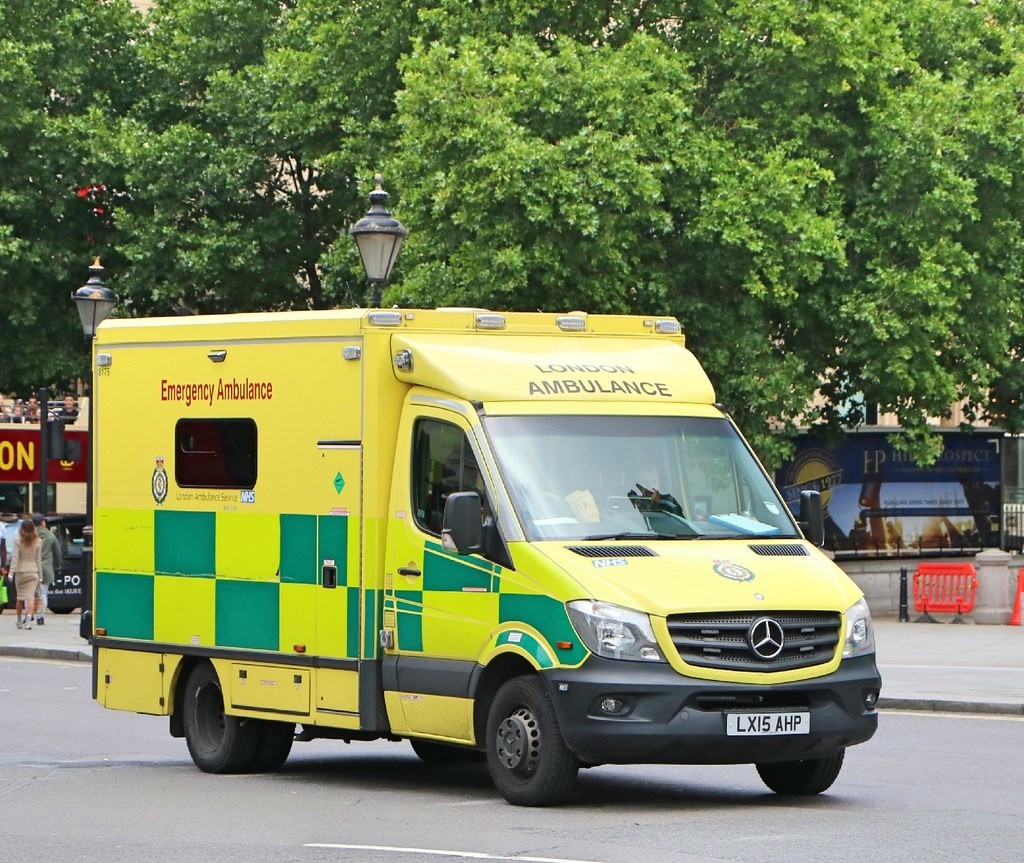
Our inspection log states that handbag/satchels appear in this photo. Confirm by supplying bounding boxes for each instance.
[0,577,8,605]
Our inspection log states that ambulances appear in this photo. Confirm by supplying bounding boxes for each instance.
[92,307,884,808]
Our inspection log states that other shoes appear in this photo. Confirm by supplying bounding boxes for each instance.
[37,616,44,625]
[22,616,35,624]
[15,621,23,628]
[24,623,31,629]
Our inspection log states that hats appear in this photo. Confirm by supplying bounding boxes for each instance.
[31,513,48,522]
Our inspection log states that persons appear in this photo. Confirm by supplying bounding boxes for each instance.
[58,393,78,425]
[0,395,25,423]
[7,519,42,630]
[0,522,6,615]
[23,514,62,625]
[24,398,38,424]
[639,465,659,491]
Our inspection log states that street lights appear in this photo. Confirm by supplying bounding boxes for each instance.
[71,254,118,640]
[349,173,409,308]
